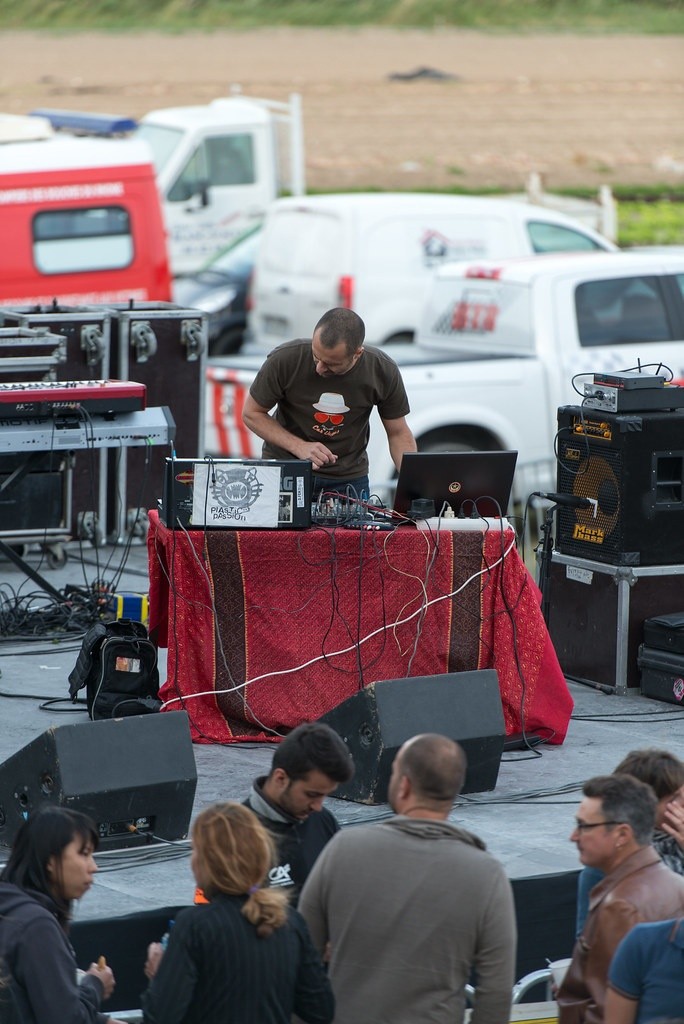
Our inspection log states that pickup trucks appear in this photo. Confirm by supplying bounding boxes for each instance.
[0,84,684,538]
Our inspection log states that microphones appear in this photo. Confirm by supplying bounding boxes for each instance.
[533,492,591,509]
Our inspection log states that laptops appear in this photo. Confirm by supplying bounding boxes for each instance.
[367,450,518,525]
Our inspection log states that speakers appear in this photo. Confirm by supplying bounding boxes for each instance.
[313,669,506,806]
[555,405,684,567]
[0,710,198,853]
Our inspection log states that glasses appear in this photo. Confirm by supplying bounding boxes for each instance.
[576,820,628,833]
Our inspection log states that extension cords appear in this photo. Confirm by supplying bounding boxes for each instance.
[417,516,508,530]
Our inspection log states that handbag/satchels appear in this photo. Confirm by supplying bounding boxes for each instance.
[68,618,159,721]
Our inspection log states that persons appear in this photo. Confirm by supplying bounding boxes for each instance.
[241,721,355,963]
[548,750,684,1024]
[139,802,336,1024]
[241,309,417,506]
[0,804,129,1024]
[297,733,517,1024]
